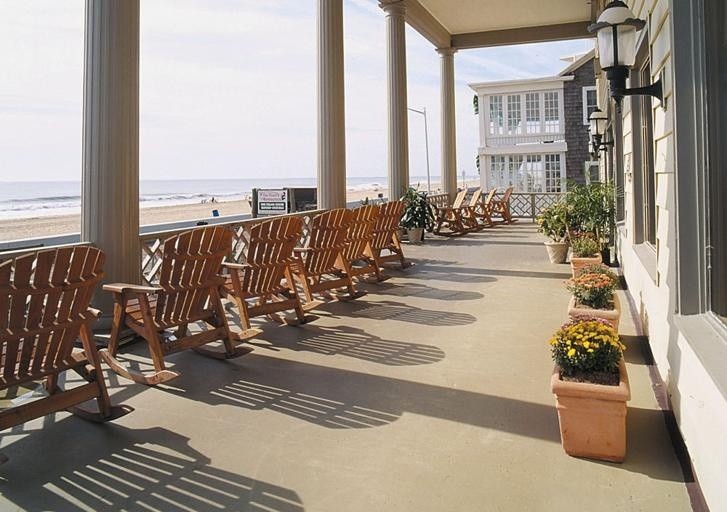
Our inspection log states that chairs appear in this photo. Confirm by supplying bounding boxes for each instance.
[367,200,408,281]
[492,187,513,224]
[0,240,112,467]
[283,208,356,311]
[98,224,235,387]
[222,214,305,331]
[461,189,482,230]
[336,205,383,292]
[434,190,468,233]
[477,188,497,227]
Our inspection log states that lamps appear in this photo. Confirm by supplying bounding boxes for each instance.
[588,0,663,108]
[587,108,614,158]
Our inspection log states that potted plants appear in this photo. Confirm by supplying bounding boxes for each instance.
[569,238,603,279]
[558,165,627,265]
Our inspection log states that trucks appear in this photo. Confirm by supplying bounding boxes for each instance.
[251,184,317,219]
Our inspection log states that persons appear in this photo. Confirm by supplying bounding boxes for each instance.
[248,195,252,207]
[245,192,247,200]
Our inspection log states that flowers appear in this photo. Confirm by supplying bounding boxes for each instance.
[562,264,619,307]
[535,203,573,242]
[549,314,626,375]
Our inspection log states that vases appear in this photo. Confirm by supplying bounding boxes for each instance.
[551,360,627,462]
[544,242,568,264]
[565,293,621,335]
